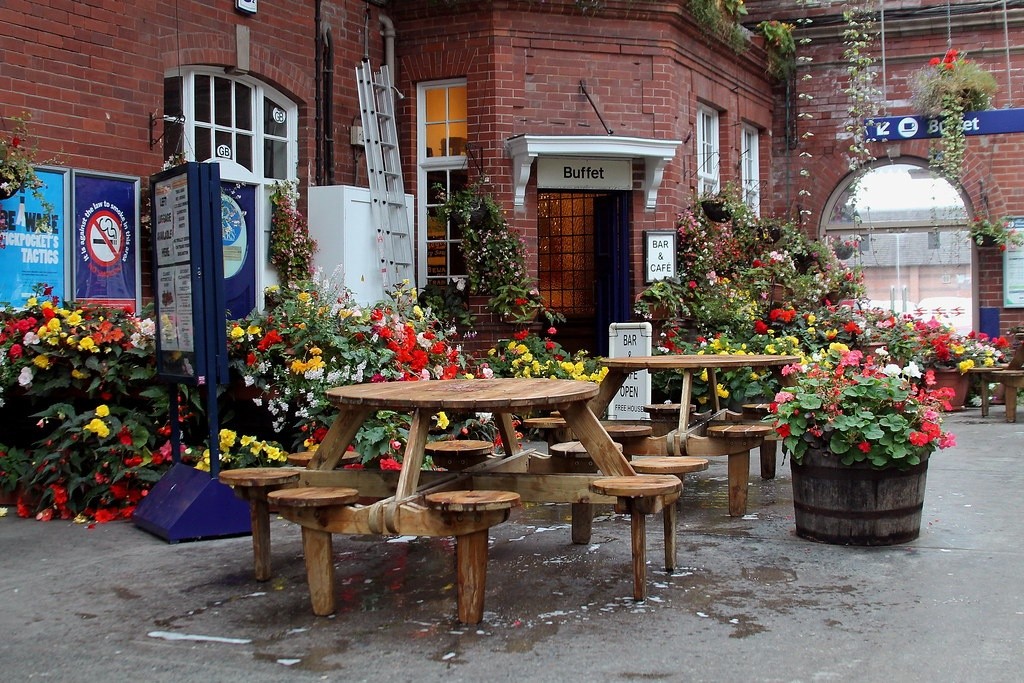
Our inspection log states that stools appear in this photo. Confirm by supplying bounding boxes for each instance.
[967,367,1024,422]
[219,404,779,624]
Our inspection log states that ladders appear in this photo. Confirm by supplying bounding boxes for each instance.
[356,56,418,310]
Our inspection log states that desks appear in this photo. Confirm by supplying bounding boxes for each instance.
[304,379,635,476]
[587,354,802,432]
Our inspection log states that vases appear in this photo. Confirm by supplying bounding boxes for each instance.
[835,247,853,259]
[858,342,887,358]
[972,231,996,248]
[701,200,731,222]
[0,178,22,200]
[757,227,782,244]
[921,367,967,406]
[993,362,1008,399]
[790,443,931,546]
[794,253,815,269]
[766,283,784,303]
[887,354,905,369]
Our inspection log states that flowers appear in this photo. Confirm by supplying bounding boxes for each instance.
[0,108,76,250]
[634,184,1024,466]
